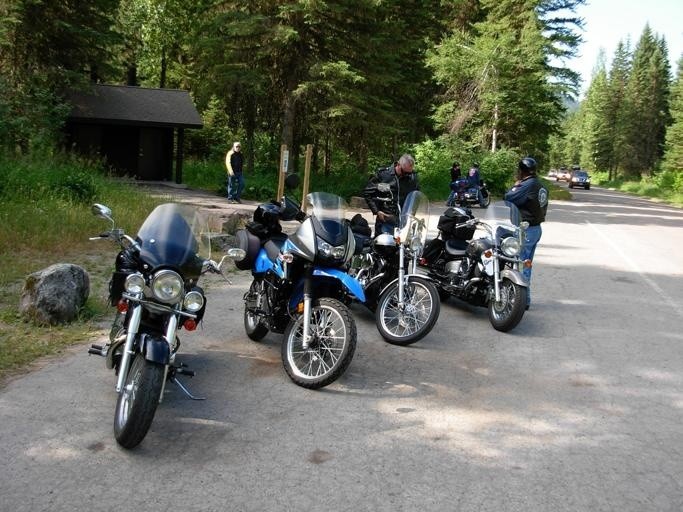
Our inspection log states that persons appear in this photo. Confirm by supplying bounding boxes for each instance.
[448,162,461,205]
[504,157,549,310]
[224,142,246,205]
[364,154,420,236]
[469,162,485,208]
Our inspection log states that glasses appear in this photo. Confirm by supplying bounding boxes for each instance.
[401,169,412,175]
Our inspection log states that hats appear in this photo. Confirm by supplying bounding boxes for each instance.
[234,143,240,147]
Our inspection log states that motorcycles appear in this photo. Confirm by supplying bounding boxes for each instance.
[87,203,247,448]
[318,182,441,347]
[446,174,491,208]
[427,200,532,333]
[231,174,388,390]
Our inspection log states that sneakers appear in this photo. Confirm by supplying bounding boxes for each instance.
[229,199,239,203]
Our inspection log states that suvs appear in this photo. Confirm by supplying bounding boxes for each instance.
[547,164,581,182]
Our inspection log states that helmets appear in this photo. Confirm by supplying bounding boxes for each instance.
[519,158,537,176]
[254,205,279,228]
[473,162,479,167]
[453,162,459,167]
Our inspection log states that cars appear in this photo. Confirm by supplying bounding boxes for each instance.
[569,170,591,190]
